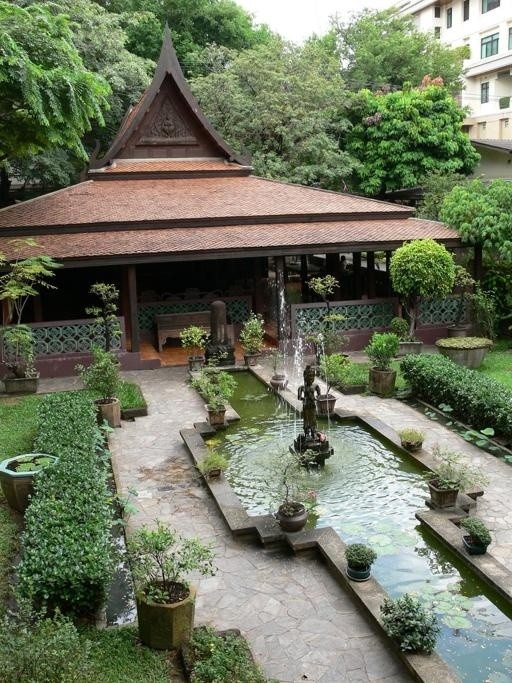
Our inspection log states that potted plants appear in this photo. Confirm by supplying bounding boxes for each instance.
[342,541,379,583]
[304,274,352,378]
[263,341,288,390]
[0,232,65,394]
[73,345,123,432]
[115,515,216,651]
[378,593,440,658]
[188,368,242,427]
[446,267,477,339]
[80,279,124,375]
[366,330,402,396]
[314,353,365,414]
[176,324,210,371]
[434,335,494,369]
[248,439,316,535]
[460,515,493,556]
[236,309,265,367]
[419,444,491,509]
[368,237,457,356]
[194,445,229,479]
[399,427,426,452]
[1,452,61,512]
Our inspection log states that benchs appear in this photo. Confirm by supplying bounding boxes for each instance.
[150,308,234,356]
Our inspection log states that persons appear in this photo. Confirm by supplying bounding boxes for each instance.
[298,364,320,441]
